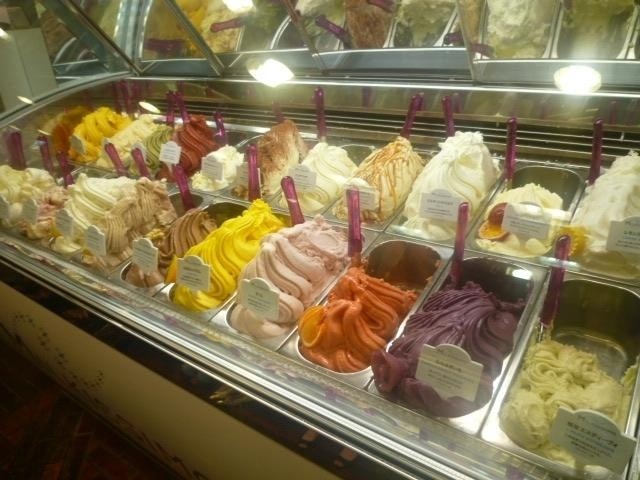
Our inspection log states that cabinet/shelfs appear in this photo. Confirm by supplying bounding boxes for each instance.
[0,0,640,480]
